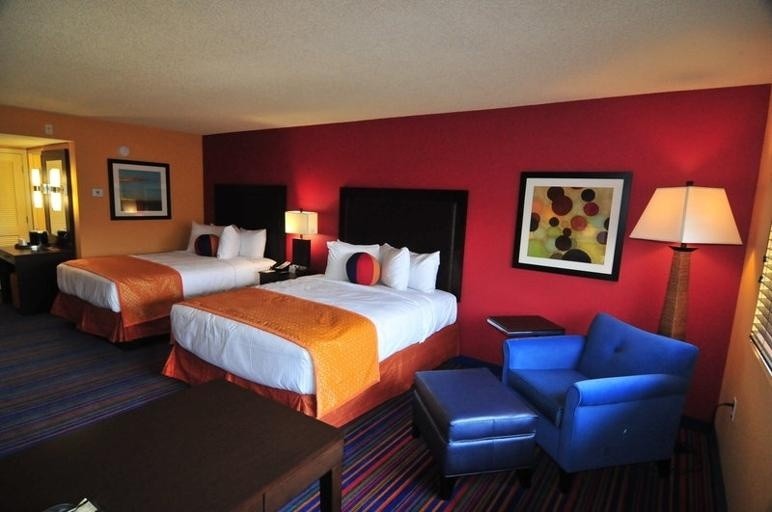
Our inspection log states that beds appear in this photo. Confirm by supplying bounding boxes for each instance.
[53,180,290,344]
[162,185,467,430]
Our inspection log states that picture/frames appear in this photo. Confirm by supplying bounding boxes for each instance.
[510,171,633,281]
[106,158,173,220]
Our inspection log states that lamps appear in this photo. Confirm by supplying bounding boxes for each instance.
[31,169,41,207]
[627,180,746,340]
[284,211,318,268]
[51,170,61,210]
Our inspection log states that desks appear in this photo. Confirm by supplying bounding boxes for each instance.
[1,377,344,512]
[1,244,59,310]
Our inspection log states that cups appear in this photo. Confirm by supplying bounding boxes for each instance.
[31,246,38,251]
[17,238,26,245]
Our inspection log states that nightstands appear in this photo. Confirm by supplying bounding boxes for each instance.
[257,269,310,285]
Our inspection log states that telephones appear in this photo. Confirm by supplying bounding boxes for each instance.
[273,259,292,271]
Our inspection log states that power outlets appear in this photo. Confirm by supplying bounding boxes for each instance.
[729,397,736,422]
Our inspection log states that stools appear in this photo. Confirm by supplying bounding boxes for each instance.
[413,368,539,479]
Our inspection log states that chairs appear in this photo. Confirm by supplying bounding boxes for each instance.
[502,306,698,475]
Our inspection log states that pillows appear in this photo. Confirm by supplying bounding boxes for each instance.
[323,239,441,291]
[187,221,266,260]
